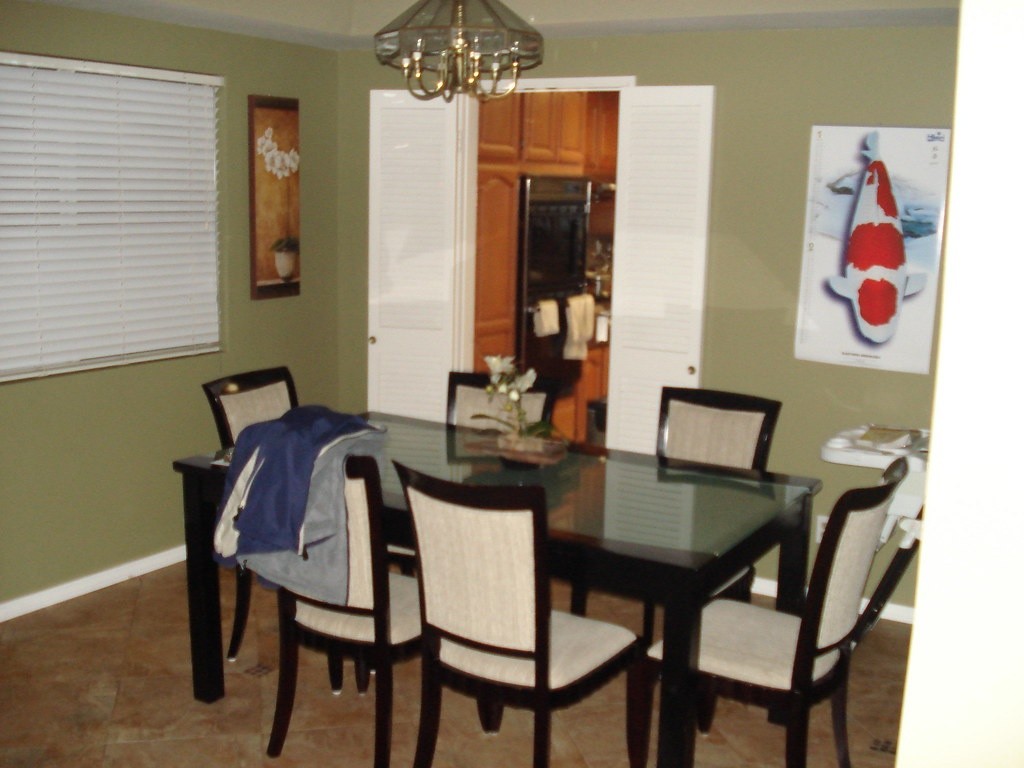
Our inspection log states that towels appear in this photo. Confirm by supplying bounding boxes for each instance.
[564,293,595,360]
[533,300,560,337]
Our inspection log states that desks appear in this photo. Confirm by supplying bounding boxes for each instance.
[172,410,824,767]
[814,426,930,640]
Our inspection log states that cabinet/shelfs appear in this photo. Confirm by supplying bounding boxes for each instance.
[474,90,619,398]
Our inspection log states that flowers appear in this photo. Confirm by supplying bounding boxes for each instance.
[468,355,568,444]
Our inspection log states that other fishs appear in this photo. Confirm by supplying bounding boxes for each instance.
[826,131,927,344]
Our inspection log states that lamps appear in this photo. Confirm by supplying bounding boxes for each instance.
[372,0,544,103]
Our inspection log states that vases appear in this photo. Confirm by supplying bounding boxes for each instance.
[495,433,545,453]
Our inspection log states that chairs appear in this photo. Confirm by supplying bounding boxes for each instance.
[631,453,909,768]
[386,458,642,768]
[382,370,558,576]
[569,384,785,735]
[263,451,490,768]
[198,362,302,661]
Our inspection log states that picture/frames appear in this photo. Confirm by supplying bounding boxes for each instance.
[247,93,300,300]
